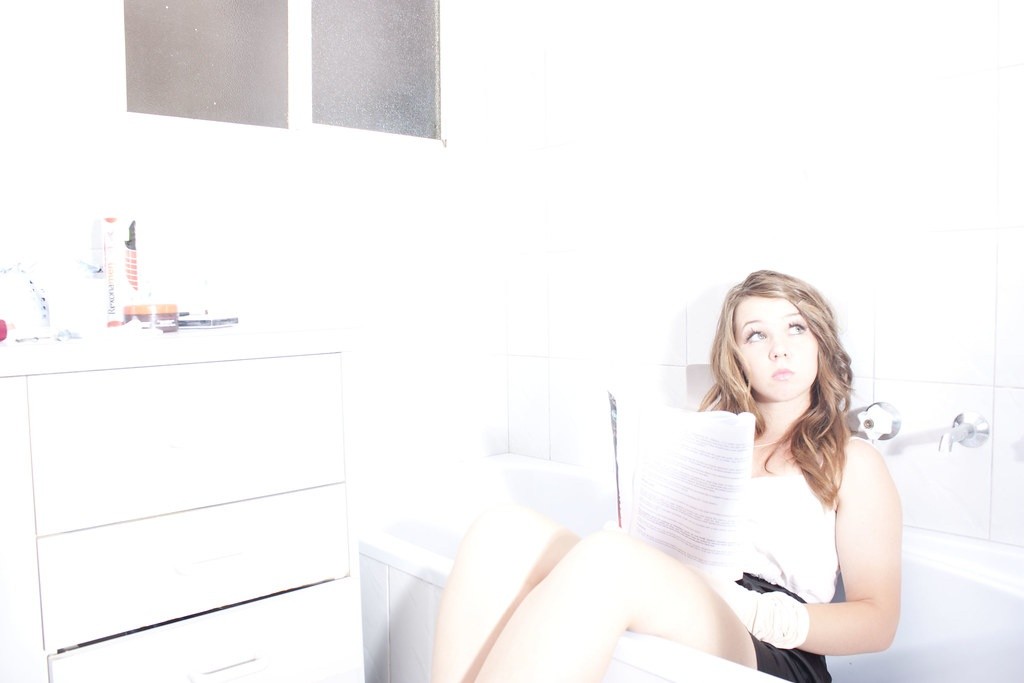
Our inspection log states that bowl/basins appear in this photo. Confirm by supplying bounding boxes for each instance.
[124,304,180,333]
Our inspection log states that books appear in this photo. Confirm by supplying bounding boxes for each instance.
[616,395,754,582]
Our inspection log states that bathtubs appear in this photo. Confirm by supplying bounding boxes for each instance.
[357,453,1022,683]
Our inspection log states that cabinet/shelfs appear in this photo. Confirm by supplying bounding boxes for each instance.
[0,328,366,683]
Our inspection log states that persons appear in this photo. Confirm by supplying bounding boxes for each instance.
[433,268,904,683]
